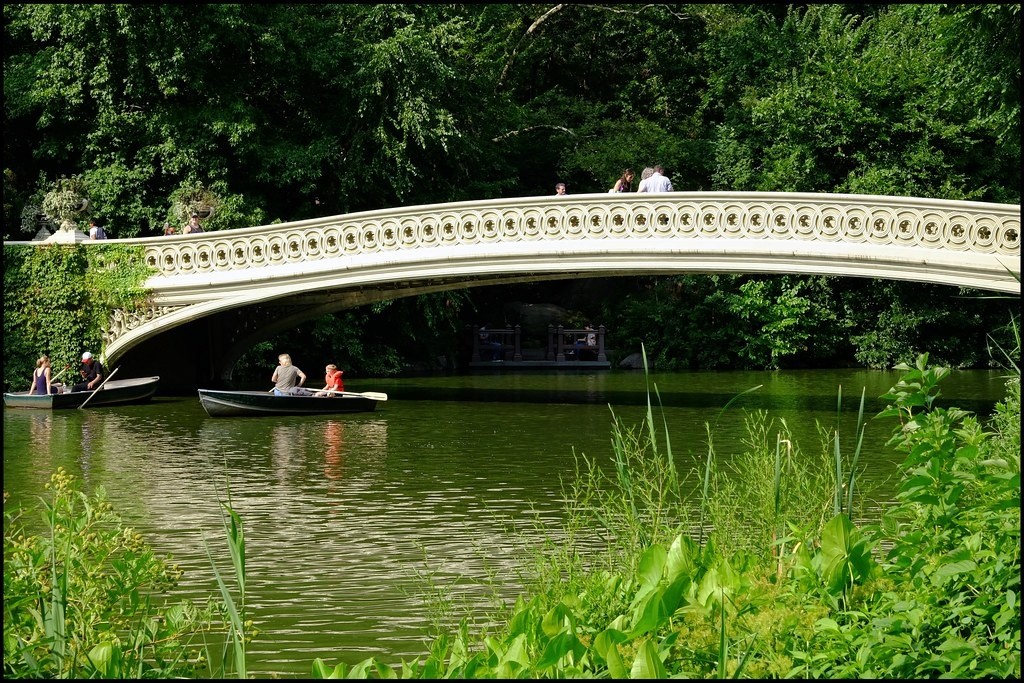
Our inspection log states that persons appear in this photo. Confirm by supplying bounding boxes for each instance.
[90,218,107,240]
[637,165,674,192]
[555,183,567,196]
[272,354,306,395]
[614,168,635,193]
[479,324,503,362]
[568,322,596,354]
[183,213,205,234]
[315,364,344,397]
[71,352,104,392]
[26,356,63,395]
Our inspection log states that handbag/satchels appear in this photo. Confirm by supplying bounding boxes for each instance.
[608,179,623,193]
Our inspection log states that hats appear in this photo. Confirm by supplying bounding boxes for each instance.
[190,212,199,218]
[81,352,92,363]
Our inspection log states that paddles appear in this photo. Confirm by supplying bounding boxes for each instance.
[300,388,388,401]
[46,365,71,386]
[77,364,123,410]
[267,386,277,394]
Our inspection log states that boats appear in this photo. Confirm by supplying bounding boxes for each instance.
[196,388,390,416]
[2,375,162,408]
